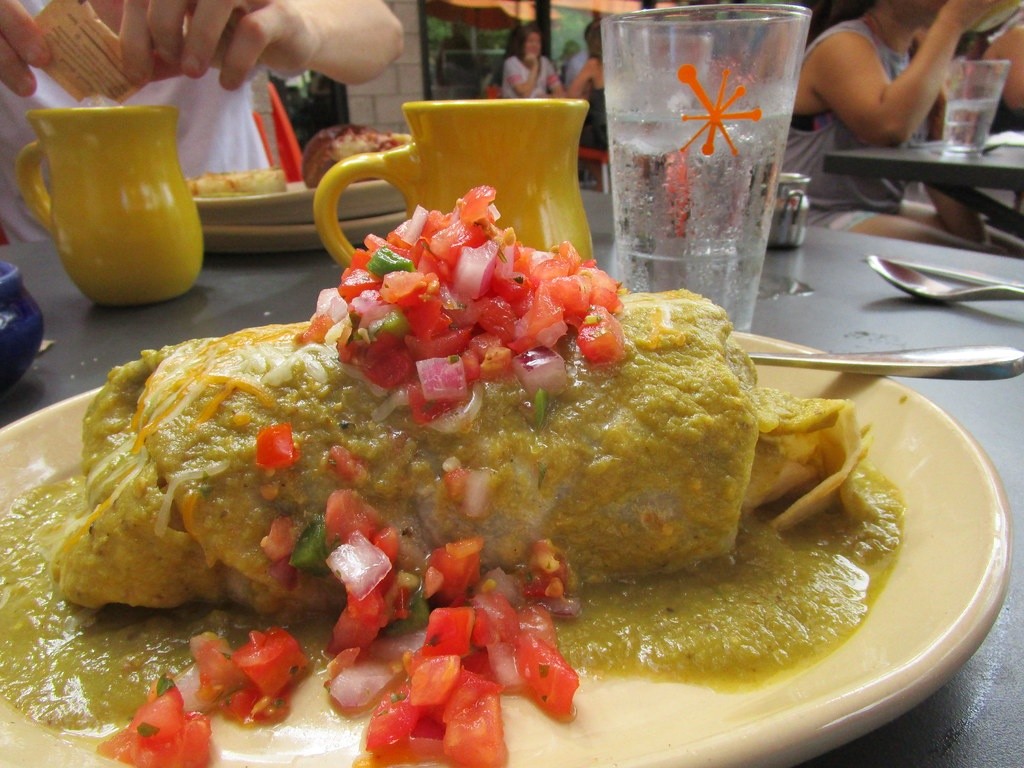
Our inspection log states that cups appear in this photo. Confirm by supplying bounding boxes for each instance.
[768,173,811,250]
[313,99,593,269]
[0,262,45,398]
[16,105,203,305]
[942,59,1012,158]
[600,2,812,333]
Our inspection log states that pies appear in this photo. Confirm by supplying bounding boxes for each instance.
[55,288,867,617]
[182,168,288,198]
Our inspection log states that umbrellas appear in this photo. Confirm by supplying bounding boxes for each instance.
[422,1,678,32]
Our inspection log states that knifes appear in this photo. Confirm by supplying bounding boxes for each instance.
[863,256,1024,290]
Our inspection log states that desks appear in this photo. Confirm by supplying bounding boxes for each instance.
[825,136,1024,239]
[0,186,1024,768]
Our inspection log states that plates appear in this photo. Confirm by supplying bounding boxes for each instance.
[0,328,1013,768]
[204,210,408,251]
[193,180,408,224]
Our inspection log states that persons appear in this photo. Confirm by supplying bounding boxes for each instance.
[775,0,1023,260]
[0,0,406,246]
[482,23,615,180]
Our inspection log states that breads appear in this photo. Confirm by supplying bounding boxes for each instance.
[300,124,413,187]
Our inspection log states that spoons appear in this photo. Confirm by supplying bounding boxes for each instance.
[867,256,1024,301]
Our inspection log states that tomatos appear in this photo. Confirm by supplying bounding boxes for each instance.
[96,183,629,768]
